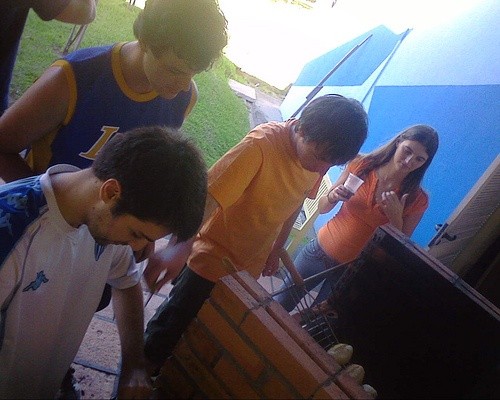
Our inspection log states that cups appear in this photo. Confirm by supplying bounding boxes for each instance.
[340,172,364,200]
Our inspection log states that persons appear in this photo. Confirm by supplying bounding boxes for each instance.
[139,94,367,369]
[266,123,439,325]
[1,127,209,400]
[0,0,97,116]
[0,0,230,183]
